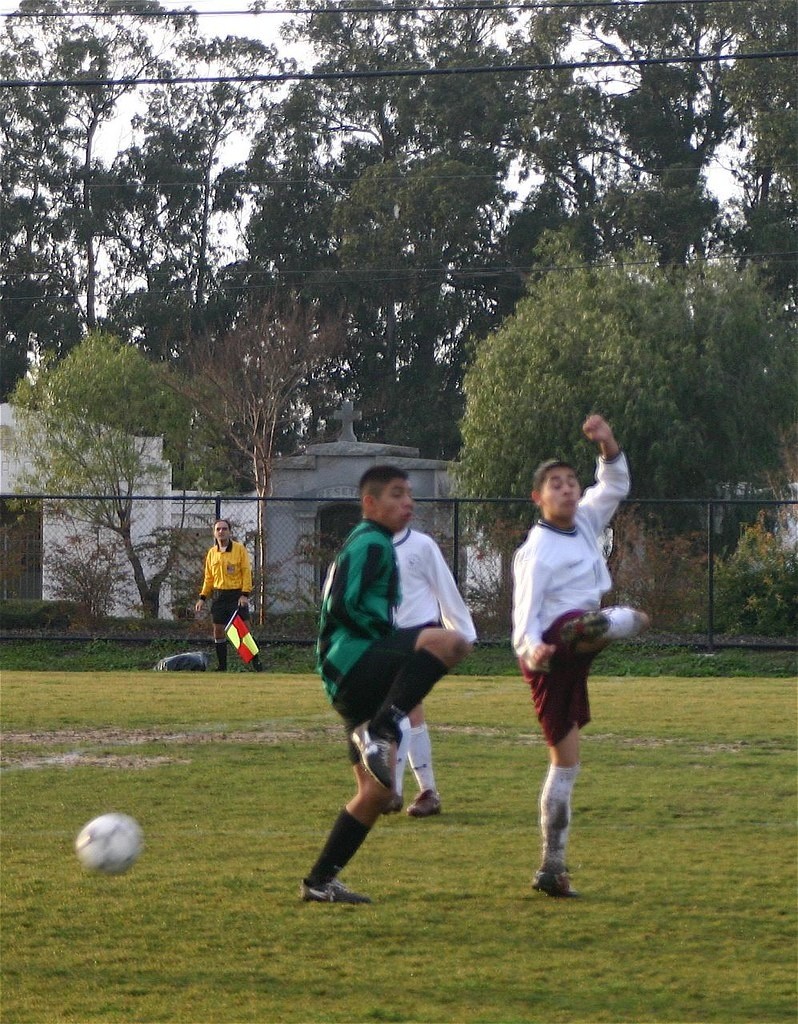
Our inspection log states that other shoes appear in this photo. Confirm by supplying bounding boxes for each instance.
[252,655,262,672]
[214,663,227,671]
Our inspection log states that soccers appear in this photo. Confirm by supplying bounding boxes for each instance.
[76,812,144,873]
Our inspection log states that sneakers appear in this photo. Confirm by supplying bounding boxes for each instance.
[381,792,403,814]
[300,877,371,904]
[348,719,395,790]
[529,871,578,897]
[406,788,441,817]
[540,610,610,646]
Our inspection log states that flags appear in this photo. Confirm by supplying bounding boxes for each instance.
[224,613,260,664]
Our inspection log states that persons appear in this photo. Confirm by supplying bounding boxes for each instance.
[321,525,478,817]
[510,414,650,897]
[195,520,263,673]
[302,465,468,905]
